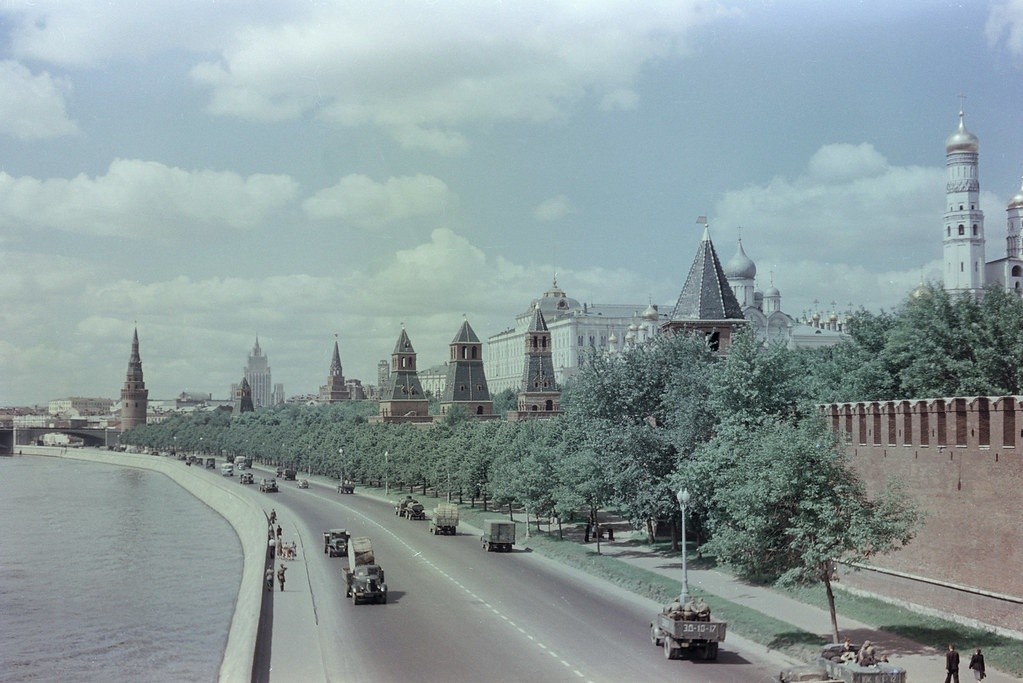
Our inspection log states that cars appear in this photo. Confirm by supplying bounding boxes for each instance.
[296,479,310,488]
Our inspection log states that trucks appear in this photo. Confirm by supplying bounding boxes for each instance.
[395,494,426,520]
[322,528,388,603]
[650,612,727,659]
[778,644,907,683]
[240,473,254,484]
[259,478,279,493]
[131,447,204,466]
[221,462,234,475]
[338,478,356,494]
[480,518,516,551]
[430,503,460,534]
[227,454,253,471]
[206,458,215,469]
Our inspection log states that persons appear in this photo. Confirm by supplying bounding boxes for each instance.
[266,565,274,592]
[269,536,276,559]
[277,539,297,561]
[839,639,889,668]
[268,509,276,539]
[944,644,960,683]
[584,522,615,542]
[667,596,711,622]
[969,649,985,683]
[277,525,282,540]
[277,564,287,592]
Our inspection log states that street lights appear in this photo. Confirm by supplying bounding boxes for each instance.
[676,486,692,606]
[308,444,312,478]
[339,448,344,485]
[384,450,389,496]
[200,436,203,455]
[173,436,177,450]
